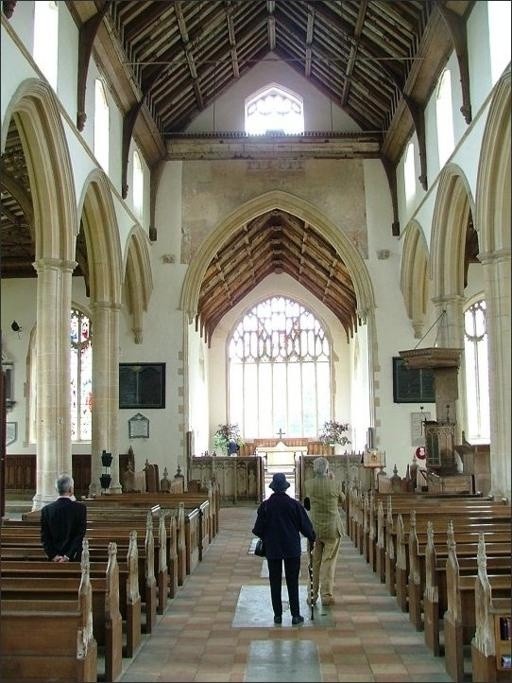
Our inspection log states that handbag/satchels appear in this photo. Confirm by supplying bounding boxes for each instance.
[254,539,266,557]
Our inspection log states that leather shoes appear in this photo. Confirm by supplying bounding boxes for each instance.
[292,616,304,624]
[274,615,282,622]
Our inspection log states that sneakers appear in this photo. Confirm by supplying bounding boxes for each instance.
[322,595,334,606]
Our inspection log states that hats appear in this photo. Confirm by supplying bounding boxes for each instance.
[269,473,290,490]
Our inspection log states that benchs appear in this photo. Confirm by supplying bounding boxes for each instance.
[340,485,511,681]
[1,482,217,678]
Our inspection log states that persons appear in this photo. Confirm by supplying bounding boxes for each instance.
[303,457,344,608]
[40,476,87,562]
[253,473,315,624]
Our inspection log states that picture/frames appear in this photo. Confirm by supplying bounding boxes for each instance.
[118,360,167,409]
[391,355,438,403]
